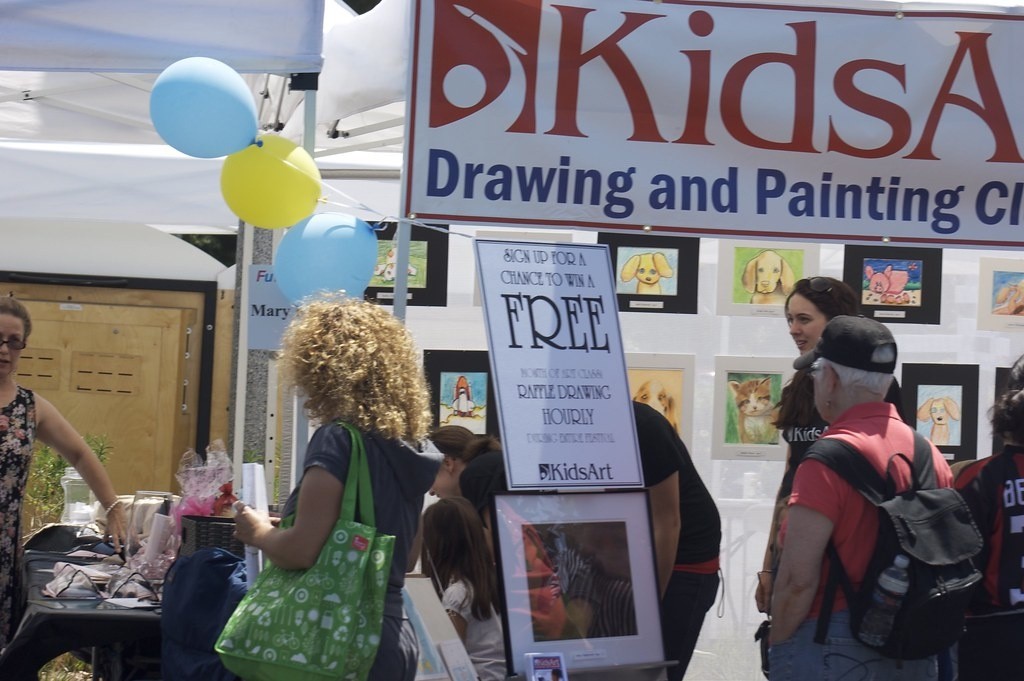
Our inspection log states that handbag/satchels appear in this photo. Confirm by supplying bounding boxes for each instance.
[213,420,396,680]
[406,574,477,681]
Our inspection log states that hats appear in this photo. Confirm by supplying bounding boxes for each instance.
[793,314,898,374]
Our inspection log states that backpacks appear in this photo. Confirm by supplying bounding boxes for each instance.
[801,431,985,661]
[159,548,248,680]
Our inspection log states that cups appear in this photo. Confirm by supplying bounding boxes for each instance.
[124,491,178,585]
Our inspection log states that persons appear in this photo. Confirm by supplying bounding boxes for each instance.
[949,355,1024,681]
[0,297,139,652]
[769,313,953,681]
[634,397,722,681]
[420,499,507,681]
[496,496,640,666]
[458,451,539,568]
[422,425,501,498]
[233,300,445,681]
[756,276,906,613]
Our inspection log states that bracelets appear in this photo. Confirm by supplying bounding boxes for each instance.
[762,570,775,573]
[104,499,123,517]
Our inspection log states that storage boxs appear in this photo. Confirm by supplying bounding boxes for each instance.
[400,577,481,681]
[523,652,569,681]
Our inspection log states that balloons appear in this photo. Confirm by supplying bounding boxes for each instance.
[272,211,379,303]
[220,134,326,228]
[148,56,263,159]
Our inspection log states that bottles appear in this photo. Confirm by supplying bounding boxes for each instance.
[857,556,909,648]
[60,467,94,526]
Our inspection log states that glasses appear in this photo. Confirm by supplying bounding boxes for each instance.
[795,278,830,293]
[0,339,25,349]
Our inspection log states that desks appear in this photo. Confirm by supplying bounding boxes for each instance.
[0,548,161,681]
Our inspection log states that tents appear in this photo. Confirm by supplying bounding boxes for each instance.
[0,0,411,226]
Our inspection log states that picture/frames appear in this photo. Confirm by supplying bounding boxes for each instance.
[624,353,693,455]
[707,355,798,463]
[993,368,1015,455]
[843,244,943,328]
[596,231,700,315]
[900,362,980,465]
[423,349,503,442]
[489,489,669,677]
[713,239,820,319]
[474,231,574,308]
[977,255,1024,334]
[363,222,450,309]
